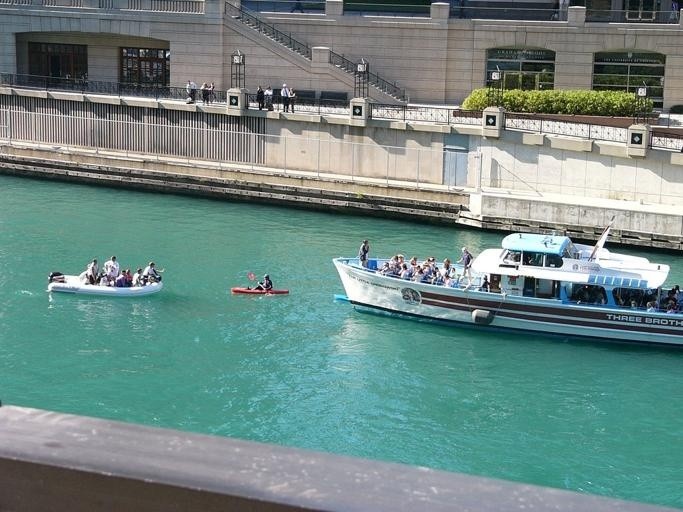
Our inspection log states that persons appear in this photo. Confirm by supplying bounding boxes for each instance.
[85,256,160,287]
[482,275,489,288]
[490,281,498,289]
[410,256,441,282]
[668,3,678,23]
[457,247,473,276]
[359,240,369,265]
[381,254,409,277]
[185,80,297,113]
[512,253,538,265]
[245,274,273,291]
[442,258,456,287]
[576,288,605,303]
[616,290,677,310]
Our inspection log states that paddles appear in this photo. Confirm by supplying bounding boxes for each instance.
[247,272,266,291]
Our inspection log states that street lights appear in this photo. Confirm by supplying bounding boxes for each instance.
[354,58,369,100]
[487,71,504,108]
[229,49,246,89]
[633,85,649,124]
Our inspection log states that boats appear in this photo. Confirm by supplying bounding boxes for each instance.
[44,273,163,299]
[230,287,289,296]
[333,215,682,349]
